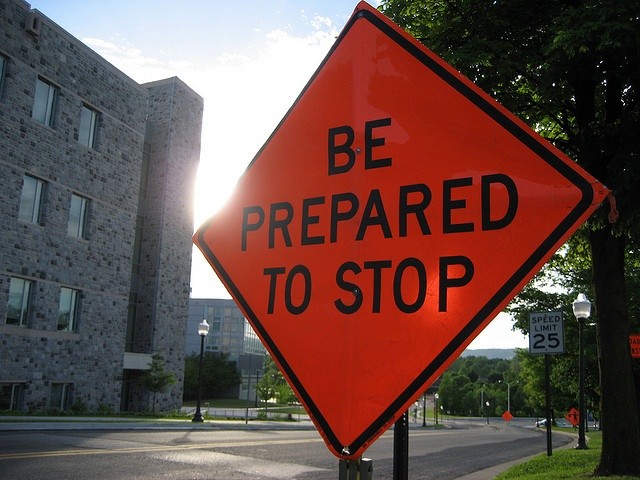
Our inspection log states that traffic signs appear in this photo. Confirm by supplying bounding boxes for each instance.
[527,310,565,353]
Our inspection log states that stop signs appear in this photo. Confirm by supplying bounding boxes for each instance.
[190,0,612,457]
